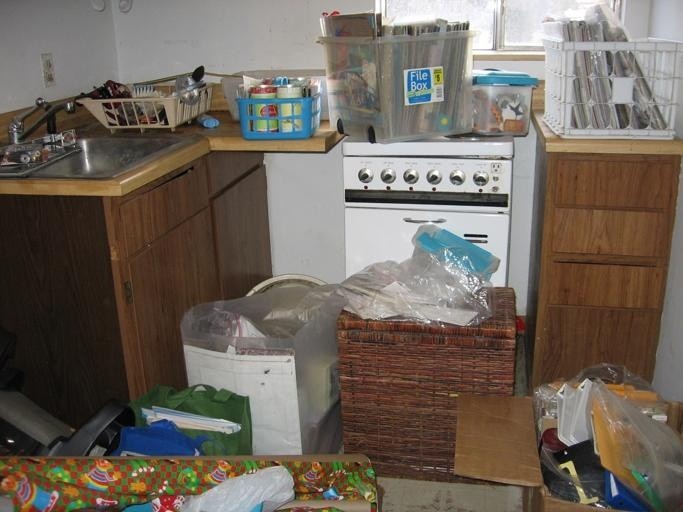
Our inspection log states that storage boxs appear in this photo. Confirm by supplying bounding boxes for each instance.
[317,29,475,143]
[471,69,538,136]
[453,394,682,511]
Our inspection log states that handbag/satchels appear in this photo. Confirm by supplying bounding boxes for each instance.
[121,384,252,457]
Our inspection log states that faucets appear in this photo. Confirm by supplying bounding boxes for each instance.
[8,98,76,143]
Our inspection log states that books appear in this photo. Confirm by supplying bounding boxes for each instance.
[543,5,669,131]
[322,12,470,136]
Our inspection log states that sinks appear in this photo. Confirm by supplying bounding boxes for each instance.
[0,134,201,180]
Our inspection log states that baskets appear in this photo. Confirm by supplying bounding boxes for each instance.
[541,37,683,139]
[237,92,322,140]
[338,287,517,483]
[76,84,212,133]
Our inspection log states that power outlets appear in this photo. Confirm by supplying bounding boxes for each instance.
[41,53,57,89]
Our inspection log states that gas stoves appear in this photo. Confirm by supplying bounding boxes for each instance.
[340,132,515,206]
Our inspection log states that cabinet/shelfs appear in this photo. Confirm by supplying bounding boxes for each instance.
[525,81,682,399]
[2,150,273,427]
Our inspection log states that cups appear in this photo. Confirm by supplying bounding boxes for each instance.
[248,83,305,134]
